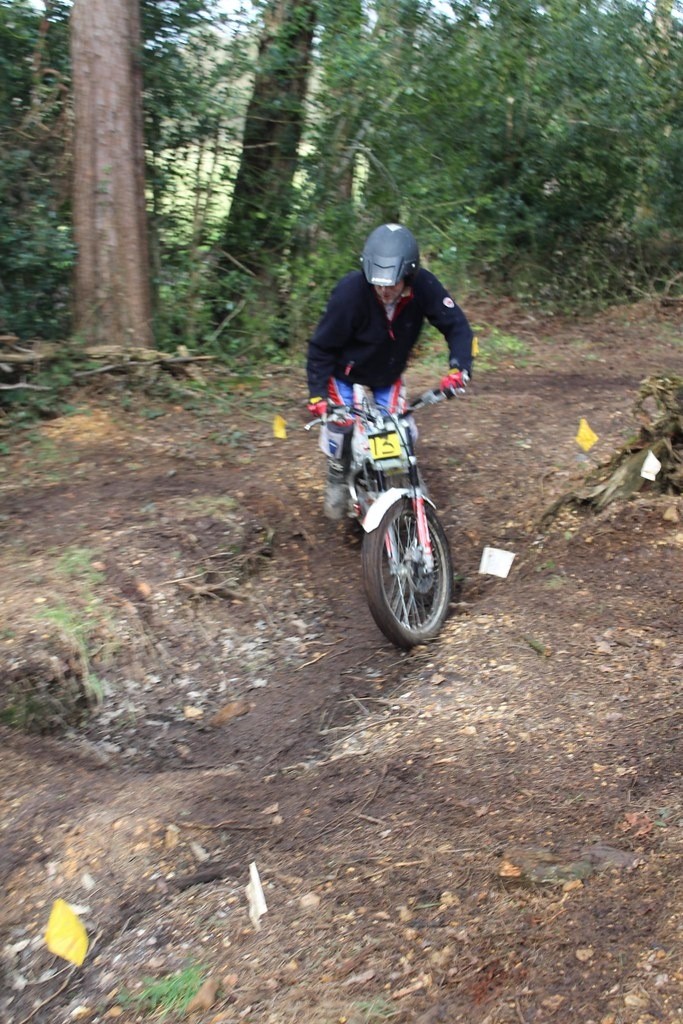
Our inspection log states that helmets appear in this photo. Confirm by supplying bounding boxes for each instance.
[363,223,419,287]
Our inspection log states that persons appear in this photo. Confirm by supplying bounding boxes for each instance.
[305,222,474,521]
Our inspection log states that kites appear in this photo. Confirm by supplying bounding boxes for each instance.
[478,546,516,579]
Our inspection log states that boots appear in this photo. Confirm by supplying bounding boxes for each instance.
[323,422,354,521]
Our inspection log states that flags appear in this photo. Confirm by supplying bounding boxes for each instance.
[44,897,90,967]
[242,861,268,920]
[272,414,288,438]
[640,450,661,482]
[471,337,479,356]
[574,418,599,451]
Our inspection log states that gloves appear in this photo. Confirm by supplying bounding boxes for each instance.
[307,396,328,418]
[440,367,471,400]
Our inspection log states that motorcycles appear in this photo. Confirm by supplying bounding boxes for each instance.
[304,373,470,648]
